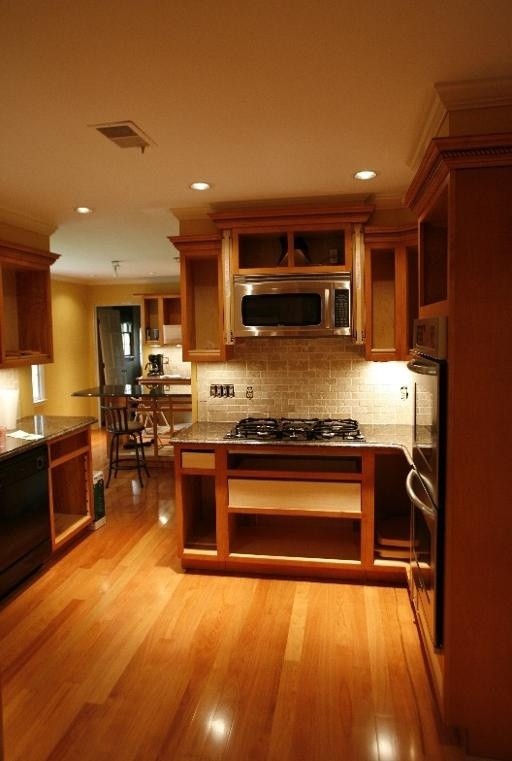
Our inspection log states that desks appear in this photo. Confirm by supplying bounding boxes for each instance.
[71,384,192,455]
[137,375,191,436]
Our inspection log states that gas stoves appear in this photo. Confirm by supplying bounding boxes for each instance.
[223,417,366,443]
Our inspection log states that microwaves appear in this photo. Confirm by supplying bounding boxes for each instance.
[231,272,353,340]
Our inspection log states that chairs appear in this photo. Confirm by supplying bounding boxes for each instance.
[130,397,170,435]
[99,405,150,488]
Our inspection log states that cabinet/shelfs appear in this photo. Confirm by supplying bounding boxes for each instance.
[179,251,227,363]
[227,449,365,571]
[48,427,95,552]
[175,445,222,562]
[0,258,54,368]
[365,242,407,361]
[231,223,353,277]
[372,452,433,571]
[140,295,181,346]
[417,174,450,317]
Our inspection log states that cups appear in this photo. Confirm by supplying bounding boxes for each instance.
[32,413,45,438]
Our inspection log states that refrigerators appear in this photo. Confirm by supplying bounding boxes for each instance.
[406,352,447,651]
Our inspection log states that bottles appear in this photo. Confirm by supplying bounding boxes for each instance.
[153,328,159,340]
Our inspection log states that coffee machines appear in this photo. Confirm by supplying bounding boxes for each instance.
[144,354,164,377]
